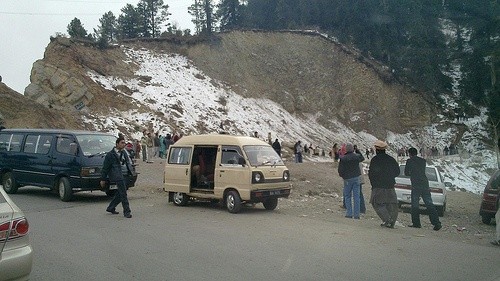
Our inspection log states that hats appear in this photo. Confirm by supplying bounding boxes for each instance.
[375,141,387,150]
[345,143,354,152]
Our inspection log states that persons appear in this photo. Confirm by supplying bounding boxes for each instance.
[294,141,357,163]
[368,141,400,228]
[405,148,441,231]
[255,131,281,156]
[100,138,132,218]
[365,144,459,157]
[126,132,184,161]
[192,147,205,186]
[337,144,366,219]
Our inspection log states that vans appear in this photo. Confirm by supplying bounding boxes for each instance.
[162,134,293,213]
[0,129,138,202]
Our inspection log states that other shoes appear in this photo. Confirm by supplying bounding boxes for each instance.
[381,218,394,229]
[124,212,132,218]
[490,240,500,246]
[408,224,421,228]
[433,224,442,231]
[106,209,119,214]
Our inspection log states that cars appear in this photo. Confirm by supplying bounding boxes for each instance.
[0,185,33,281]
[479,169,500,224]
[394,163,452,216]
